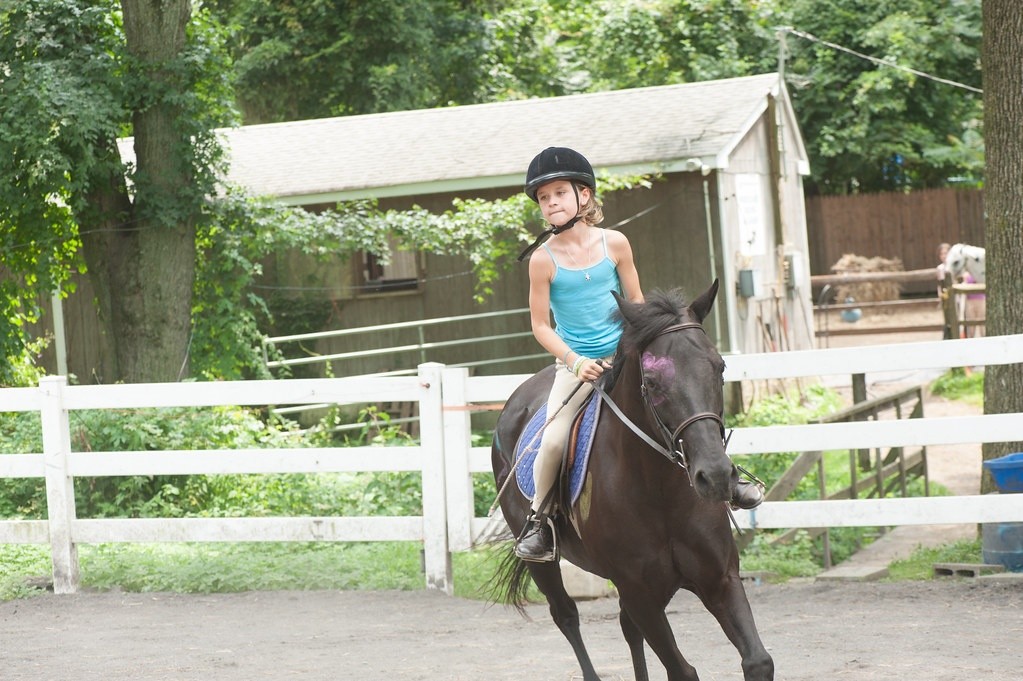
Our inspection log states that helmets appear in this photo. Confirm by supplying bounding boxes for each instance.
[524,147,596,203]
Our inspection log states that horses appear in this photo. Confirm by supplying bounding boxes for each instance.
[475,278,775,681]
[944,243,986,284]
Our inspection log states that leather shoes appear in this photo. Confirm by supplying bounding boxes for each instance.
[730,479,763,509]
[516,521,552,558]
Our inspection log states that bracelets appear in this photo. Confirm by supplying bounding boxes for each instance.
[563,349,590,379]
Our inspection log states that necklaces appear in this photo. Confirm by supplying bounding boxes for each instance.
[556,226,591,281]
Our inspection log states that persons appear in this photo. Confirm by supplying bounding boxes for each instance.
[935,242,986,339]
[513,146,765,559]
